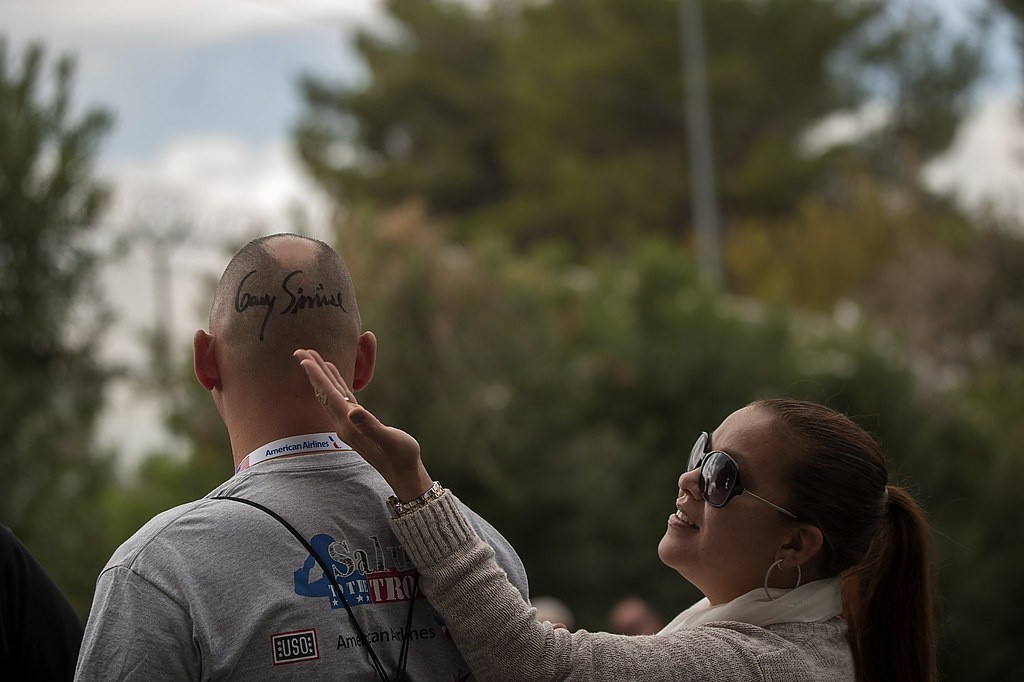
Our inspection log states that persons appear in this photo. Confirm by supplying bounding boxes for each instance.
[532,598,661,635]
[0,521,84,682]
[293,349,936,682]
[74,234,529,682]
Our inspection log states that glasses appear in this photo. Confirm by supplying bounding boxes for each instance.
[688,431,793,518]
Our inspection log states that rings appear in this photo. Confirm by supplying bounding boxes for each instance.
[345,397,349,401]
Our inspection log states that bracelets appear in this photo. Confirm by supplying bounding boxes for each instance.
[386,483,443,518]
